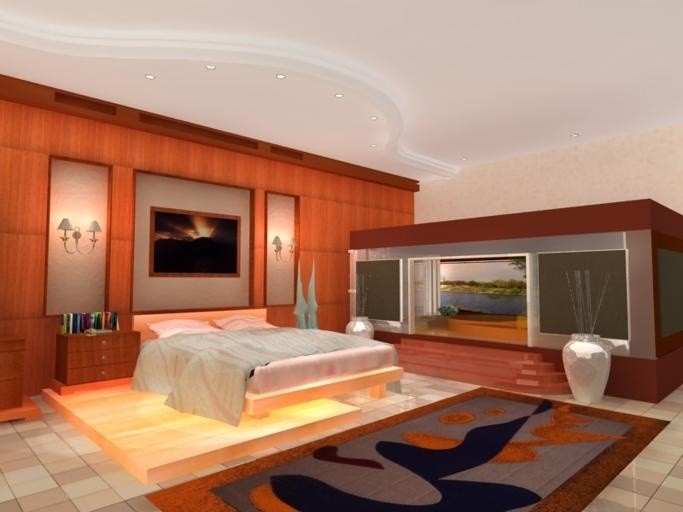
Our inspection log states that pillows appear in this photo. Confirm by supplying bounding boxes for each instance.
[146,319,222,339]
[212,316,272,332]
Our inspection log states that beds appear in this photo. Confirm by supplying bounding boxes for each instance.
[133,308,403,420]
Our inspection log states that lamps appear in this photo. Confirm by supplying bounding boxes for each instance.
[271,236,297,264]
[56,218,101,255]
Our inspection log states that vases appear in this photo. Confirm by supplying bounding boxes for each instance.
[561,333,612,404]
[345,316,374,340]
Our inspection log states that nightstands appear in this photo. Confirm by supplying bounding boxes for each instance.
[0,332,25,411]
[55,329,140,385]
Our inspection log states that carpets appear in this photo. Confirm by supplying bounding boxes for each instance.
[142,387,670,512]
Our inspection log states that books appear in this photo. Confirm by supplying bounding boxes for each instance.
[60,310,120,334]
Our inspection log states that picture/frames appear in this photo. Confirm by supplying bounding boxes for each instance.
[148,205,241,278]
